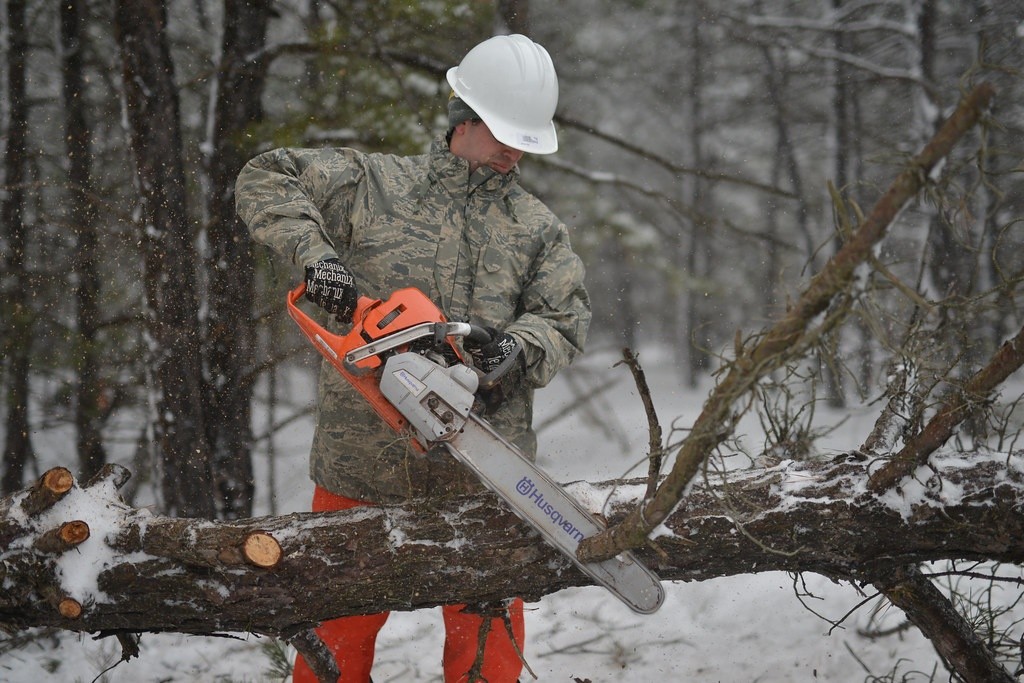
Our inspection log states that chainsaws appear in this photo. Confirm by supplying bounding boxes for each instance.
[286,282,667,615]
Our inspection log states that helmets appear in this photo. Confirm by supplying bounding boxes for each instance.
[446,34,558,155]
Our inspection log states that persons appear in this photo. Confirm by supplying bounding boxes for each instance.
[233,33,591,683]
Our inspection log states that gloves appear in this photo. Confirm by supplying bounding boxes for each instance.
[466,326,517,374]
[304,258,358,325]
